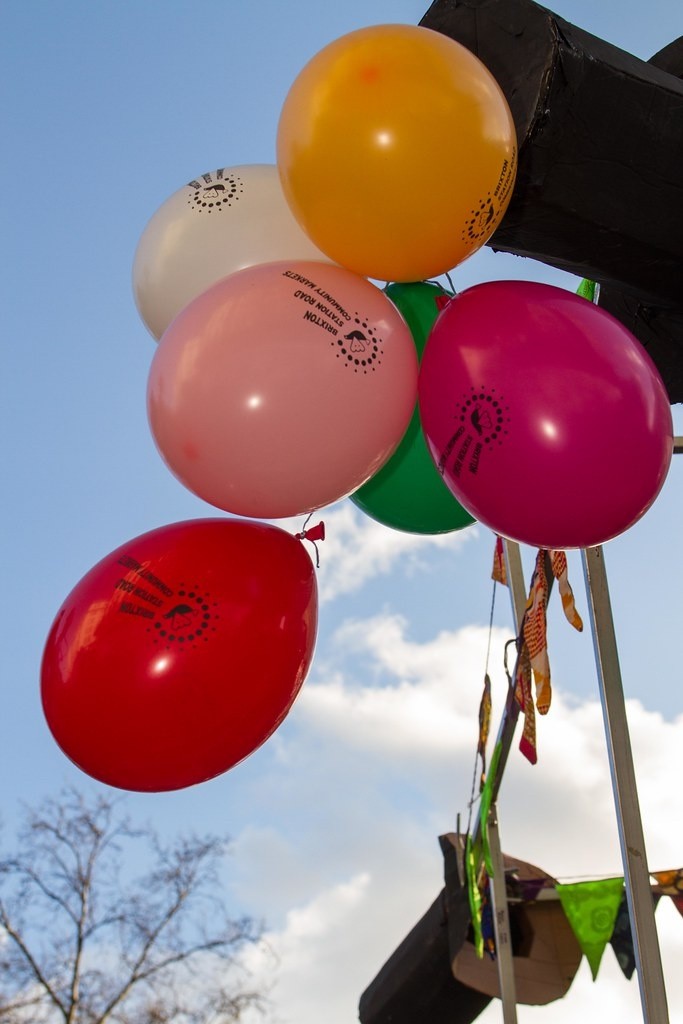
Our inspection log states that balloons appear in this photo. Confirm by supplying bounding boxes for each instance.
[39,515,326,795]
[145,261,416,521]
[419,277,673,548]
[342,281,478,535]
[133,165,346,340]
[276,22,519,285]
